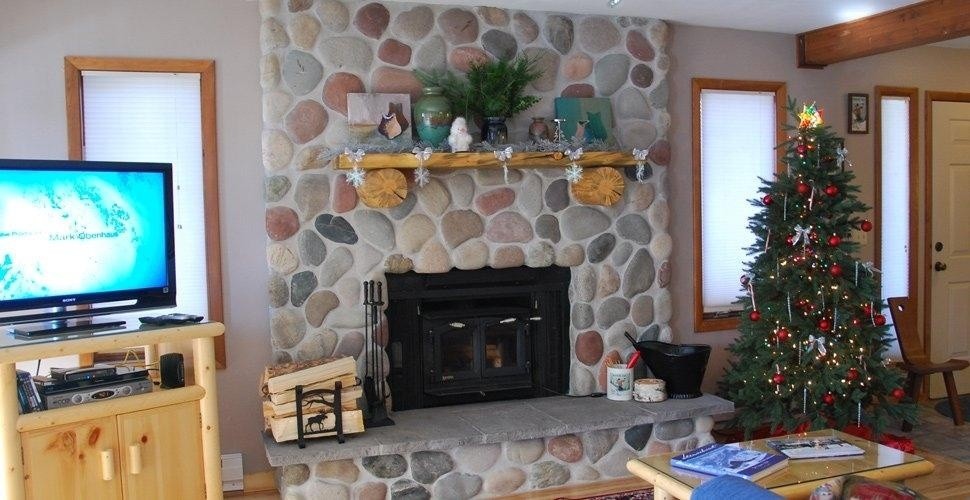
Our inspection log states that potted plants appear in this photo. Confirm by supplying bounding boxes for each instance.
[413,49,545,149]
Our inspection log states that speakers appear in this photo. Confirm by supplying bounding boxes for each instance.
[160,353,185,389]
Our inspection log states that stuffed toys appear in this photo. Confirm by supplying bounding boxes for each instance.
[448,116,474,154]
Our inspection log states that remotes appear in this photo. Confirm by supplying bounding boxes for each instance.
[138,313,204,326]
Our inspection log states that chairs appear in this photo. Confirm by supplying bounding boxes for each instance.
[888,297,970,432]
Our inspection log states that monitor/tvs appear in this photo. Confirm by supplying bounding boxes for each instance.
[0,158,177,336]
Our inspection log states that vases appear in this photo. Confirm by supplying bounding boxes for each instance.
[528,118,549,146]
[411,88,452,153]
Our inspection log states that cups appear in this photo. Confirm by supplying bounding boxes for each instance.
[606,364,634,403]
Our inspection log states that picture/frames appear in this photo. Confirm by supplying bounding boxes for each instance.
[848,93,869,134]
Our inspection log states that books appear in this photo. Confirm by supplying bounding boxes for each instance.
[766,435,866,460]
[669,441,791,485]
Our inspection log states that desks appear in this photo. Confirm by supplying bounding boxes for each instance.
[627,429,934,500]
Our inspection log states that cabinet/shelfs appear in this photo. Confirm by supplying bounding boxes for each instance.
[1,319,223,500]
